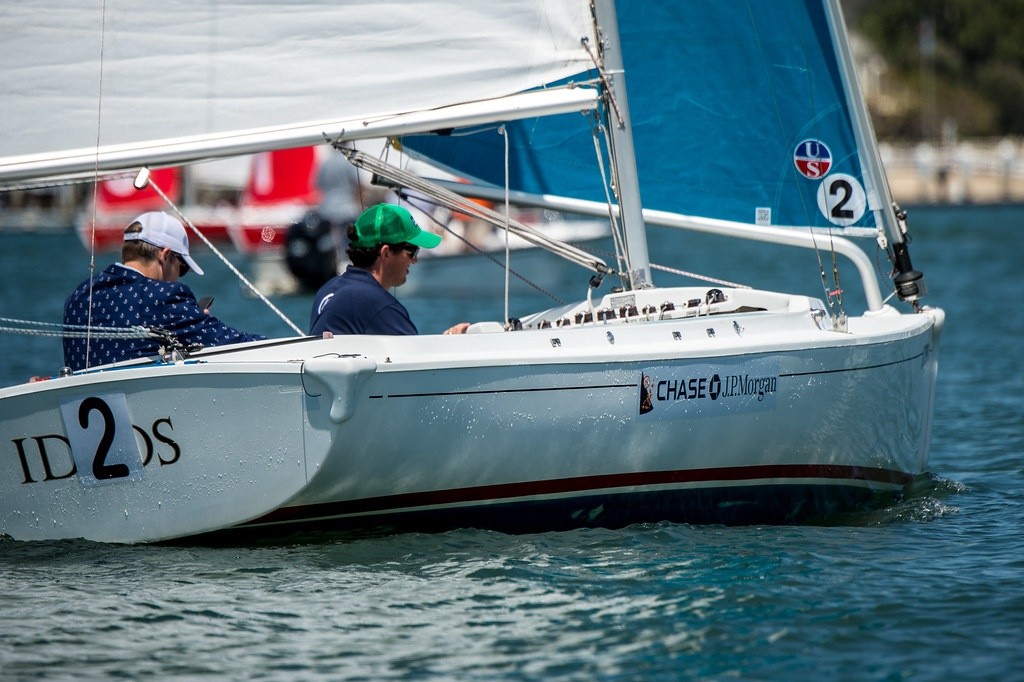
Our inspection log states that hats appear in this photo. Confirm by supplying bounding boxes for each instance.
[123,211,204,276]
[352,202,442,249]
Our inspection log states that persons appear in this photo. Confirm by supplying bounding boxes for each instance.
[63,212,268,371]
[309,203,470,335]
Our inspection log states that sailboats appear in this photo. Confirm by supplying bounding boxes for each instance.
[1,0,948,547]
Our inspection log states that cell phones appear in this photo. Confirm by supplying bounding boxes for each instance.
[199,297,214,309]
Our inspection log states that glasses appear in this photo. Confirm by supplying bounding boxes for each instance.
[161,248,190,277]
[379,243,419,260]
